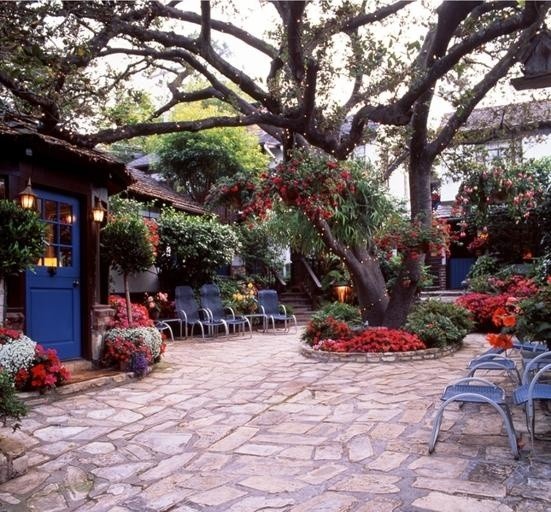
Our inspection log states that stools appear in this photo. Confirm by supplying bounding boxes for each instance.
[424,335,551,464]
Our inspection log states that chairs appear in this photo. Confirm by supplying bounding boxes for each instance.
[200,283,255,341]
[155,322,175,340]
[257,289,300,334]
[172,283,231,341]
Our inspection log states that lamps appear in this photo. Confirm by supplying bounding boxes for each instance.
[39,257,60,266]
[18,175,37,209]
[333,278,350,302]
[93,195,106,223]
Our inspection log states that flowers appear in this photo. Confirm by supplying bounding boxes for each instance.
[100,321,166,376]
[0,326,73,394]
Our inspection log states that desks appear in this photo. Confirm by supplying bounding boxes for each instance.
[157,317,183,343]
[230,312,269,333]
